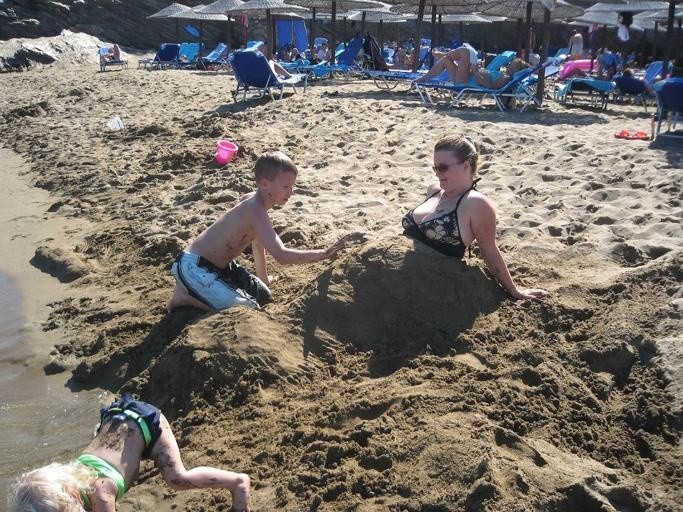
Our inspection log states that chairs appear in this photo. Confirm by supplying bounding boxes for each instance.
[649,79,682,142]
[644,60,674,106]
[137,39,616,113]
[98,47,128,71]
[613,75,657,115]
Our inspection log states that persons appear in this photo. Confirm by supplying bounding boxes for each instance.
[397,132,550,304]
[5,391,250,512]
[99,32,683,118]
[164,150,354,313]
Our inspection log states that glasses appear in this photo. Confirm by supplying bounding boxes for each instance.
[431,160,465,173]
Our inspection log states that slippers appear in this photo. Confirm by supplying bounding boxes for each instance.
[632,131,650,141]
[614,130,633,139]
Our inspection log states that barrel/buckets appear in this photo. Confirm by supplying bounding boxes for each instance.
[215,140,238,164]
[106,116,124,131]
